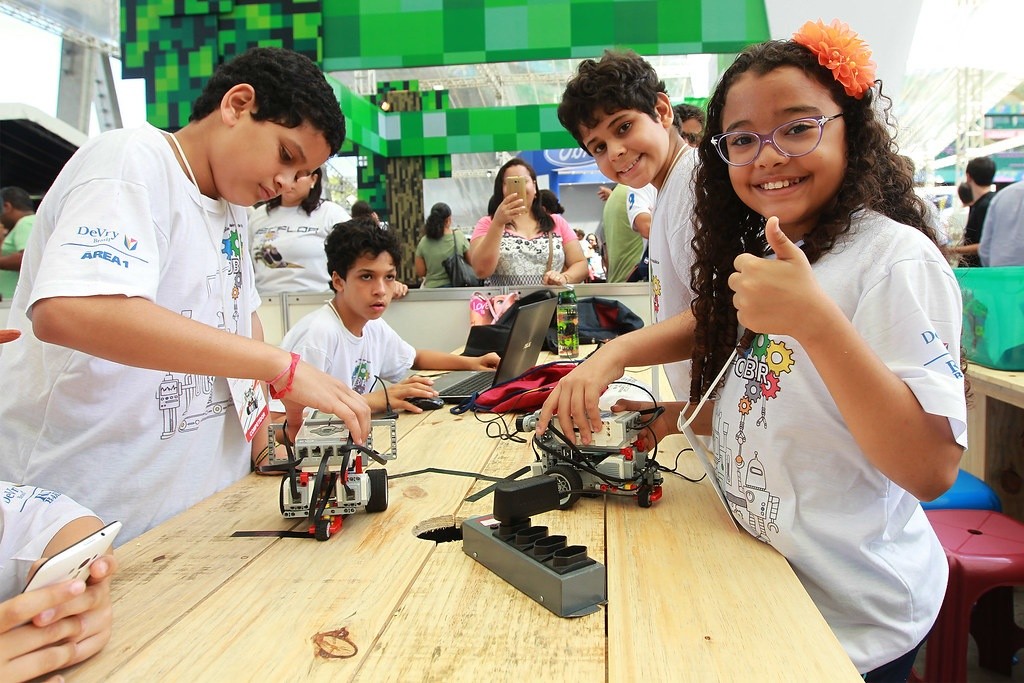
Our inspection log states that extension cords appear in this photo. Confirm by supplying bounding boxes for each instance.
[461,513,608,618]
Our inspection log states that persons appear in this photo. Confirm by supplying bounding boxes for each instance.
[0,481,118,683]
[242,103,706,426]
[608,19,967,683]
[893,154,1024,269]
[0,186,36,301]
[1,47,424,549]
[535,50,701,444]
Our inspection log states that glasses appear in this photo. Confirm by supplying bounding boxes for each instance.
[680,131,702,145]
[710,113,845,167]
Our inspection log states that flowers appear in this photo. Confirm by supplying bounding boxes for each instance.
[792,17,876,99]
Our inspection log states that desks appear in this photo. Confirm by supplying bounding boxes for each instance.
[958,360,1024,512]
[30,344,865,683]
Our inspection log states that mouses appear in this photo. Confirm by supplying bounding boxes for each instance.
[405,397,444,412]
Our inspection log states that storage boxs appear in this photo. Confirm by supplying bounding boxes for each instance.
[950,266,1024,373]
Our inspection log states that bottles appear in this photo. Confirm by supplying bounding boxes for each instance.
[557,286,579,358]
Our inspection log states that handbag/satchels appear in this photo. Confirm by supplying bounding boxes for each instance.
[470,291,518,324]
[442,230,480,287]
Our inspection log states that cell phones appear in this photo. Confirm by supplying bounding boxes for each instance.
[506,176,528,213]
[20,520,122,594]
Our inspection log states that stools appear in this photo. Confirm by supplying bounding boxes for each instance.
[907,509,1024,683]
[919,468,1002,510]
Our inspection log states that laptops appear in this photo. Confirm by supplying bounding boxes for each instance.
[406,297,559,403]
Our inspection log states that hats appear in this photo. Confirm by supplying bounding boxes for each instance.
[460,325,512,357]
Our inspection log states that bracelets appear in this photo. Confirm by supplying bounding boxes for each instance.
[265,357,293,386]
[270,352,301,398]
